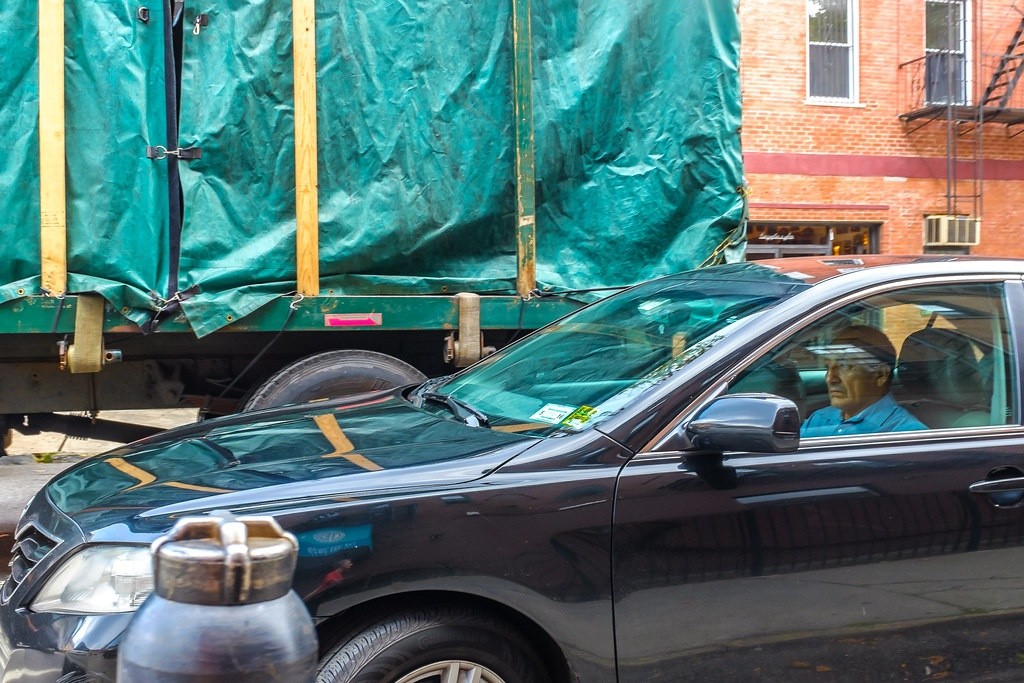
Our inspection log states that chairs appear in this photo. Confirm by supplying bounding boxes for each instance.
[897,327,991,433]
[729,352,808,429]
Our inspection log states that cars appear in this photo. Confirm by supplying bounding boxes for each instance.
[0,255,1024,683]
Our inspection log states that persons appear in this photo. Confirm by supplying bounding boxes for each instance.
[799,325,927,439]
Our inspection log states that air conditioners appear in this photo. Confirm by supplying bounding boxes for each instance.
[924,216,981,246]
